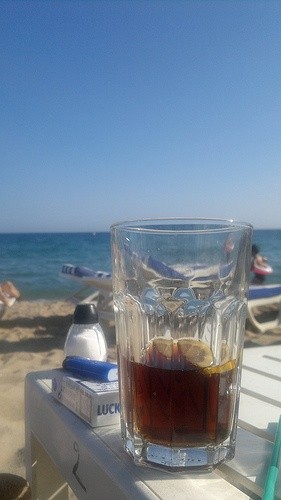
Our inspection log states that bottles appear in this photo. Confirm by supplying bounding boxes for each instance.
[64,303,106,361]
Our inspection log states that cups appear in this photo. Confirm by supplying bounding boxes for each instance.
[109,218,254,474]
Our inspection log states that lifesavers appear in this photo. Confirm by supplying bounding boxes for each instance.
[252,263,273,275]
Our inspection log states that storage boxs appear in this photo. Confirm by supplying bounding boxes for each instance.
[50,376,120,427]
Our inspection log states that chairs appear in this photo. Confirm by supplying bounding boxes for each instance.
[60,254,281,334]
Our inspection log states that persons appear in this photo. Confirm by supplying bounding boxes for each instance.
[251,245,267,284]
[0,281,21,314]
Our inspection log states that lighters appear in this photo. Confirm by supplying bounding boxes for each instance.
[62,356,119,382]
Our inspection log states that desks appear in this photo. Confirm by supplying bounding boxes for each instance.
[25,346,281,500]
